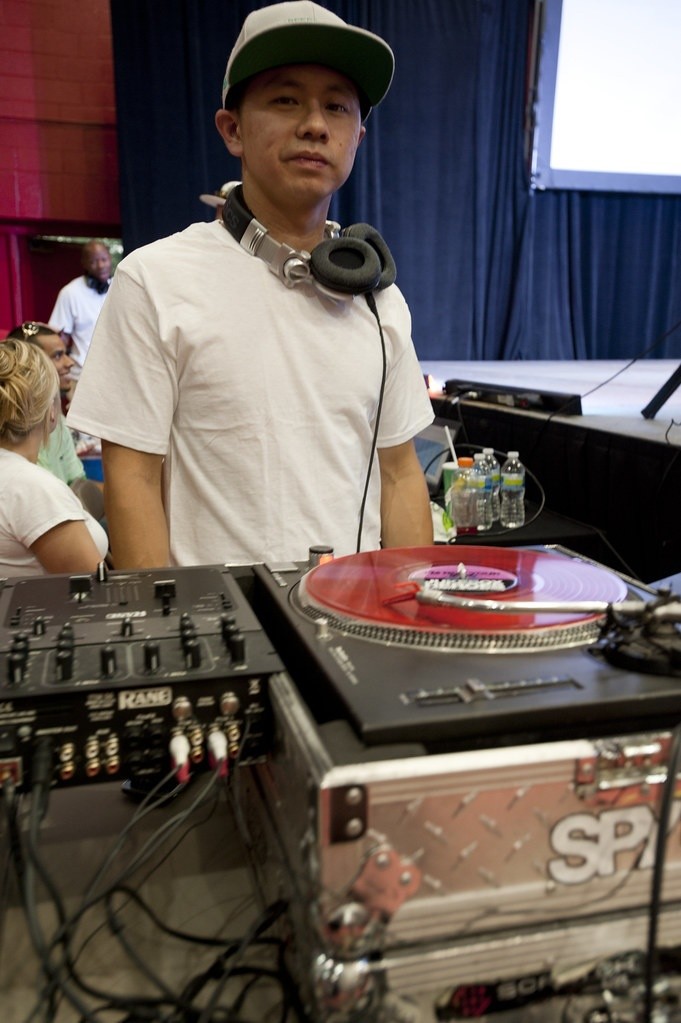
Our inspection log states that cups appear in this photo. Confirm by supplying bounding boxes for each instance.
[441,461,459,514]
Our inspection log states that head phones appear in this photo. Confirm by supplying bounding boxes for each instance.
[85,270,111,295]
[222,182,396,296]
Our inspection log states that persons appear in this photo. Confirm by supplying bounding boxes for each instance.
[7,324,86,486]
[48,241,115,400]
[0,339,109,579]
[63,1,439,573]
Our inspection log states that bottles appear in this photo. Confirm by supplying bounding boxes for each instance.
[451,457,478,536]
[501,451,525,528]
[483,448,501,522]
[472,453,493,530]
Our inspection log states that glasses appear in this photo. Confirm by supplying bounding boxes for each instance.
[22,320,41,341]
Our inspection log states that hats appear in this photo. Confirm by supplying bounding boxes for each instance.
[222,0,396,113]
